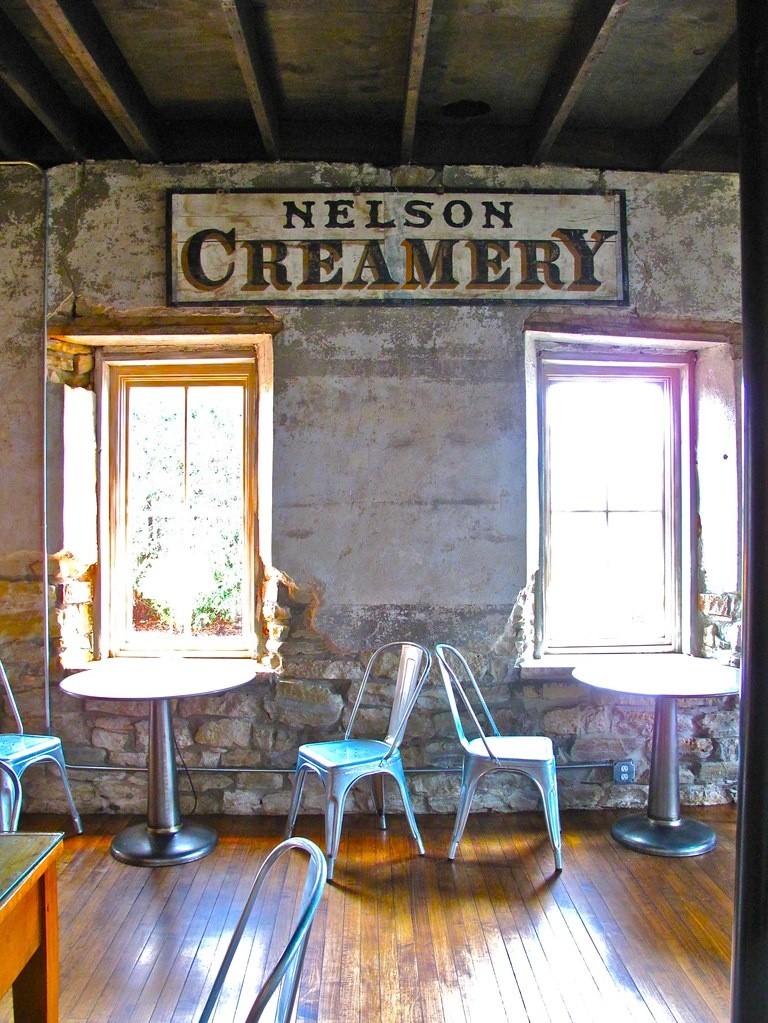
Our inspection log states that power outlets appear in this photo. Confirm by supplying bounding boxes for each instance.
[613,760,636,784]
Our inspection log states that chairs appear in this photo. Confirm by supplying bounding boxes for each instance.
[435,642,562,871]
[285,640,432,879]
[197,837,328,1023]
[0,661,84,836]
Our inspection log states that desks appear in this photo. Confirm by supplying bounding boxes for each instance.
[0,831,64,1023]
[59,659,256,867]
[571,659,741,856]
